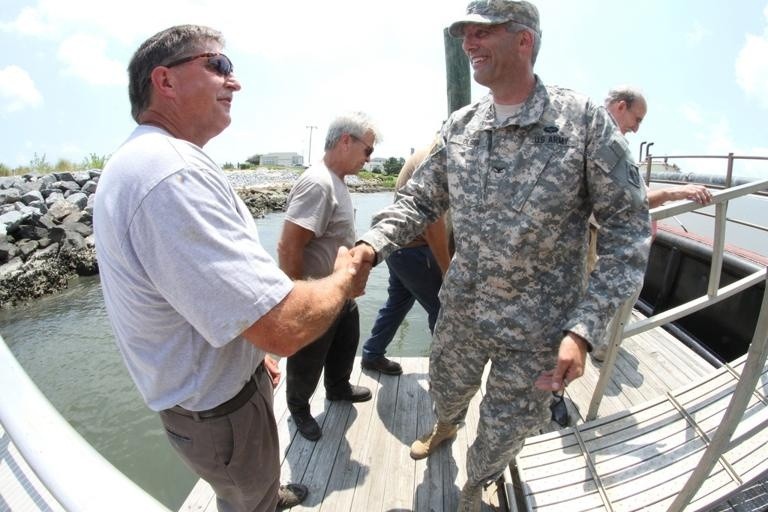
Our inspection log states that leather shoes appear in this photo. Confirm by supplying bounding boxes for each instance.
[291,412,322,441]
[325,383,372,401]
[360,356,403,375]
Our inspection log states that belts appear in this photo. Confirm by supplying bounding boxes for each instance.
[166,358,265,420]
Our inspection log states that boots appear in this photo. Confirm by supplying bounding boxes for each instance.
[457,479,482,512]
[410,419,459,460]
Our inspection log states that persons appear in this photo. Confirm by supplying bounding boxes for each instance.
[92,25,369,512]
[277,110,378,440]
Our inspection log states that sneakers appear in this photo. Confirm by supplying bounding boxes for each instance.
[277,484,308,509]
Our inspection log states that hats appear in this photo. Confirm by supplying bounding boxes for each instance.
[447,0,541,39]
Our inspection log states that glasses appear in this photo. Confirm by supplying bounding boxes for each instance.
[550,388,568,428]
[350,135,374,156]
[165,52,234,74]
